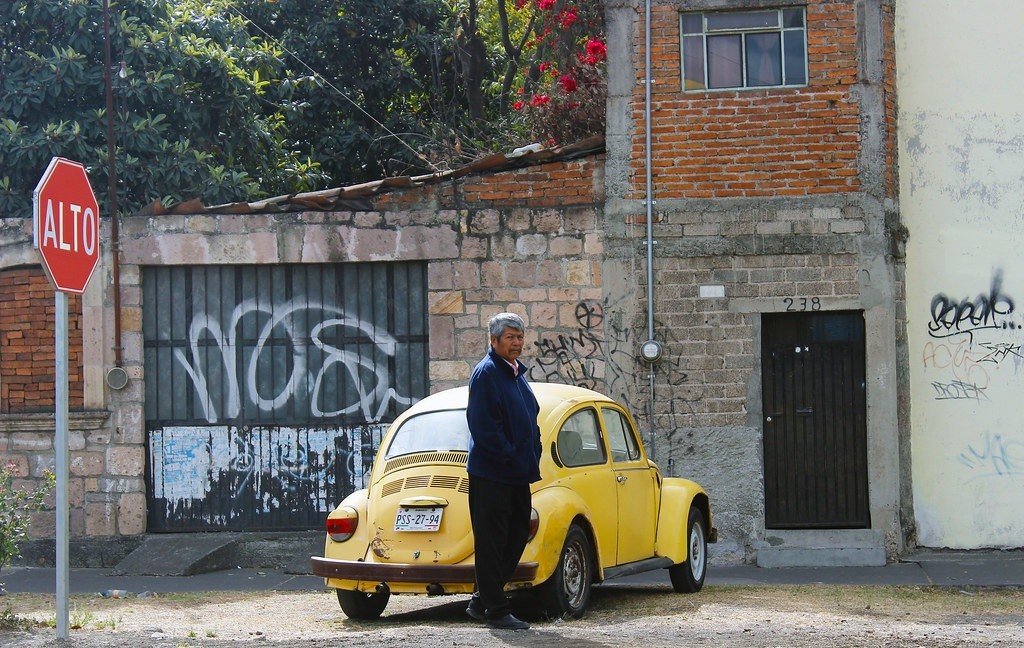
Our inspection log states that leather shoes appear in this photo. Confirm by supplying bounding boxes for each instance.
[466,600,484,619]
[486,612,530,629]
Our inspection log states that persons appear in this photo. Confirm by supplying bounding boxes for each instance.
[464,311,543,628]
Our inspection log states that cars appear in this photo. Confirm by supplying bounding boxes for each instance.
[311,382,718,619]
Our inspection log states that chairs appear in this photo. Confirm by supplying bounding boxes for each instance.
[558,431,584,465]
